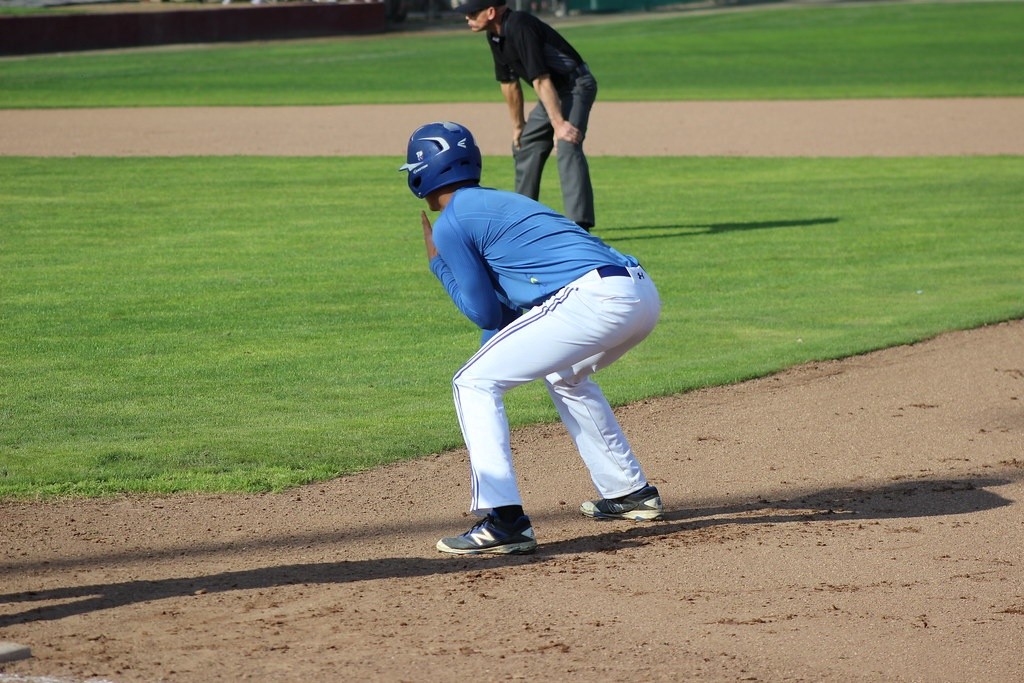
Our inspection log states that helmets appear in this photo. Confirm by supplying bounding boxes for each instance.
[399,122,482,198]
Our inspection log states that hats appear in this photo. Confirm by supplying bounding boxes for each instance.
[454,0,507,13]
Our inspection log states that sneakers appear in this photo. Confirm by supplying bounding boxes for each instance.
[435,513,537,555]
[579,486,664,521]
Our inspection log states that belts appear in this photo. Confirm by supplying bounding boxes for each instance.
[597,265,631,279]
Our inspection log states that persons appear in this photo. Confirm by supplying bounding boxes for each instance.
[453,0,597,235]
[398,120,665,554]
